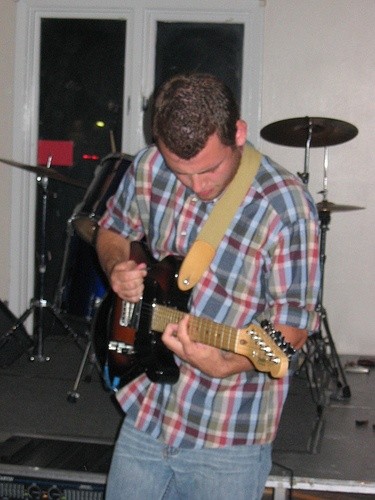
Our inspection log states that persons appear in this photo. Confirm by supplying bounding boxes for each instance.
[95,74,321,500]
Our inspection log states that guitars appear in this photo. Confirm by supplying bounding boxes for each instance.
[109,241,296,384]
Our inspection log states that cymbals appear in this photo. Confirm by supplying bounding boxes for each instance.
[259,116,359,148]
[0,158,88,189]
[316,202,366,213]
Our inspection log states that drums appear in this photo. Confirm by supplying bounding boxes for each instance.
[68,153,148,245]
[93,289,112,371]
